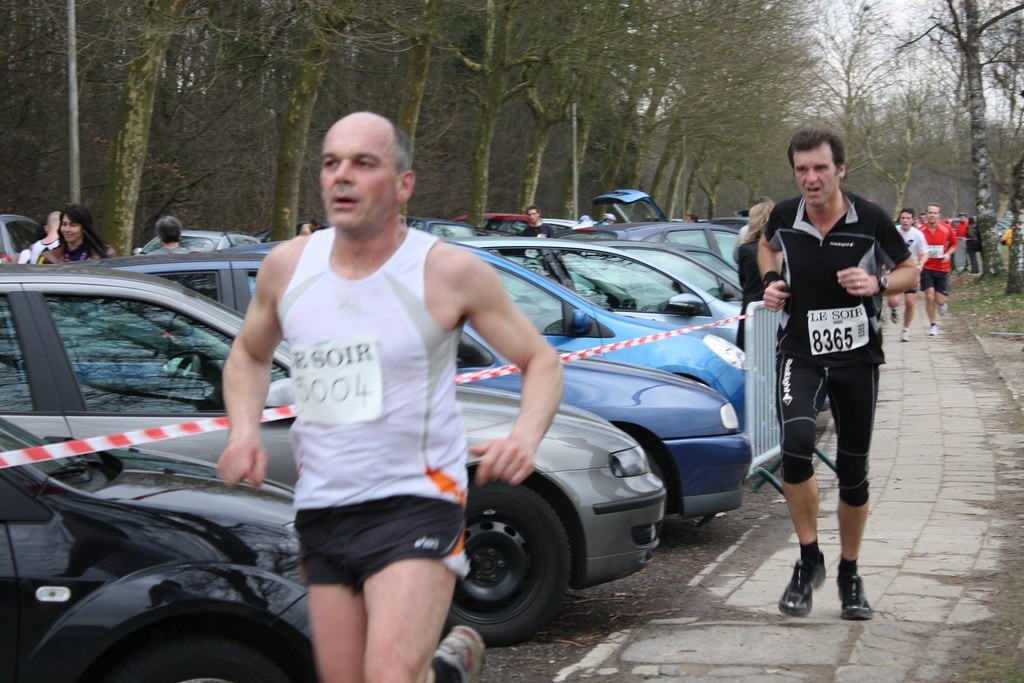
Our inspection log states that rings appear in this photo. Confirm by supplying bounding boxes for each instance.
[858,281,861,288]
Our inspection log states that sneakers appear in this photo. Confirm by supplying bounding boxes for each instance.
[837,574,872,619]
[434,624,485,683]
[779,553,825,616]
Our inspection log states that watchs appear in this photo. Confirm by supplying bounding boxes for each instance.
[874,275,889,296]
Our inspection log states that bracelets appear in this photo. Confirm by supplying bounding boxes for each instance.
[762,270,781,287]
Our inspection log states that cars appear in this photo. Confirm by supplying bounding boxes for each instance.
[950,214,1014,240]
[62,249,755,550]
[0,189,747,440]
[0,417,318,682]
[0,262,667,649]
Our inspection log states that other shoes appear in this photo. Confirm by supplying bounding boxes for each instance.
[901,330,909,341]
[938,303,948,315]
[929,326,937,336]
[890,308,899,324]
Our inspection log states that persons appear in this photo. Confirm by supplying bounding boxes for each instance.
[888,207,929,341]
[300,220,318,235]
[596,213,617,226]
[687,214,699,224]
[217,111,564,682]
[145,216,190,255]
[757,128,920,619]
[521,205,555,239]
[734,196,787,353]
[894,212,1012,274]
[918,203,958,336]
[10,204,116,264]
